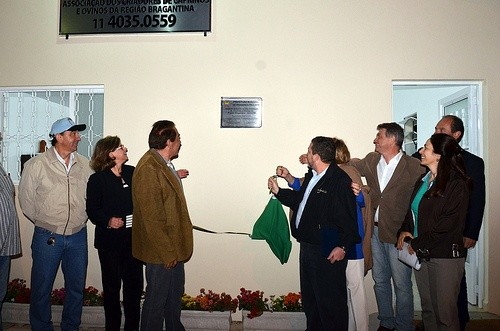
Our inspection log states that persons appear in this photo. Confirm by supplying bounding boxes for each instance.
[0,162,23,331]
[86,136,189,331]
[18,117,96,331]
[131,120,194,331]
[268,115,485,331]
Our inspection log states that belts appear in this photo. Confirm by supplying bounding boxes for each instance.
[374,222,378,227]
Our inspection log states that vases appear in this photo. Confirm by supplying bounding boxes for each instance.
[1,303,306,331]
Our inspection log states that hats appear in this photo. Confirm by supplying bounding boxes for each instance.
[49,117,86,141]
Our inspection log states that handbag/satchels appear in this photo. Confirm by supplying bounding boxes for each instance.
[251,195,292,265]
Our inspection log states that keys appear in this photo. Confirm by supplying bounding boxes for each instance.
[47,237,55,246]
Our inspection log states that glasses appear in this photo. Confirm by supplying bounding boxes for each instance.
[114,144,125,152]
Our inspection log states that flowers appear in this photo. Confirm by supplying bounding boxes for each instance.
[2,279,304,319]
